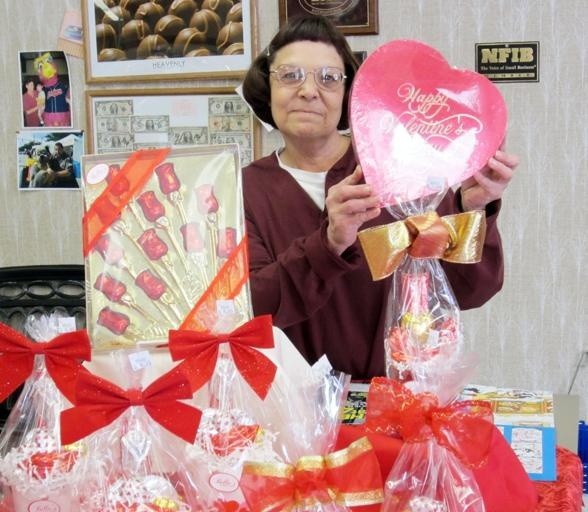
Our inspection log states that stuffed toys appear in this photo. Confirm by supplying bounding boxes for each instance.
[33,52,71,126]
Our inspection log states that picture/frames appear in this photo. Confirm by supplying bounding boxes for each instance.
[278,0,379,36]
[85,88,264,169]
[81,0,261,83]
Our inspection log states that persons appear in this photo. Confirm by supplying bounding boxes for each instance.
[33,83,46,125]
[23,142,73,187]
[23,82,41,127]
[241,14,519,378]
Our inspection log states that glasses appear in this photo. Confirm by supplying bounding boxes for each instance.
[271,63,350,92]
